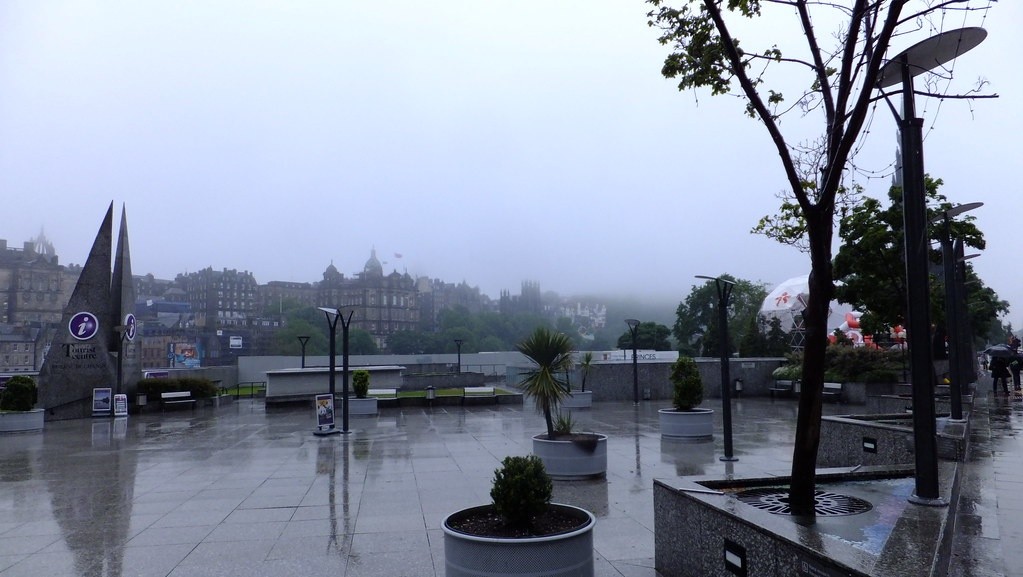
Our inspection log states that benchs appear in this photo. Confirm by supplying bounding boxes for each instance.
[159,390,197,414]
[461,387,499,405]
[767,379,792,399]
[822,382,843,404]
[365,388,401,407]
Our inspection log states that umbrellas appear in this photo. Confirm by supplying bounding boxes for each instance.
[996,343,1013,350]
[983,346,1014,360]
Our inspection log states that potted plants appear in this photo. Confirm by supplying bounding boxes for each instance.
[557,352,596,409]
[0,375,46,433]
[515,325,608,481]
[340,367,378,416]
[657,353,714,440]
[438,455,596,577]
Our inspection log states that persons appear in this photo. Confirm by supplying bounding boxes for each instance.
[1010,350,1023,391]
[989,357,1011,399]
[982,354,989,369]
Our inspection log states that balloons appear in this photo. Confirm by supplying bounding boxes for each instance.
[891,326,907,350]
